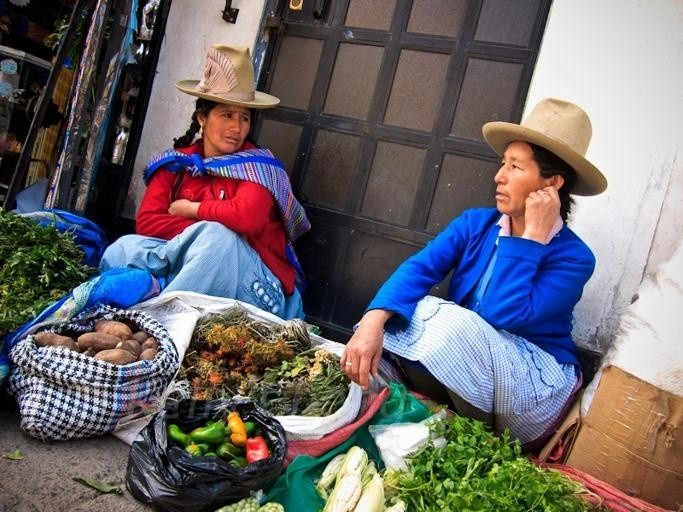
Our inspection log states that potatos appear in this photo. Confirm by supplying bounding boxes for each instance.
[33,318,158,366]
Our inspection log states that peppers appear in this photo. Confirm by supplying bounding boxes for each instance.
[167,406,272,469]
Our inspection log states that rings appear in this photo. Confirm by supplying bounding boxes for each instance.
[345,361,351,367]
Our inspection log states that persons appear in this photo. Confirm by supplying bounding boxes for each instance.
[98,45,312,321]
[340,97,607,452]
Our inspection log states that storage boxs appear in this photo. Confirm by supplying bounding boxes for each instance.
[538,365,683,511]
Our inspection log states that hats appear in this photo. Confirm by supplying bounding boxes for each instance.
[174,44,280,108]
[482,98,608,196]
